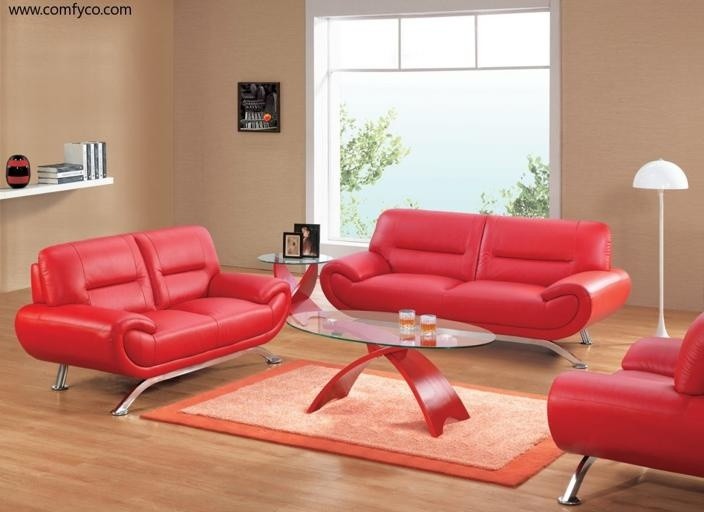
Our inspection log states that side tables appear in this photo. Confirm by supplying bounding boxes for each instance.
[257,251,335,322]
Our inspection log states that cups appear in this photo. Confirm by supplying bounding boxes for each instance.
[399,331,417,346]
[419,313,437,337]
[399,309,415,335]
[420,335,437,348]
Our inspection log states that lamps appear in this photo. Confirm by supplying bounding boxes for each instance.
[632,158,689,339]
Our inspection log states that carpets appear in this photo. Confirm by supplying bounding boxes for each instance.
[140,358,565,489]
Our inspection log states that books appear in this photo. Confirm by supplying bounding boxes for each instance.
[36,141,106,185]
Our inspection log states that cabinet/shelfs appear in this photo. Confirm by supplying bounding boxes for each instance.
[0,176,114,202]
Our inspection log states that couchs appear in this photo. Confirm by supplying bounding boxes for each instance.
[319,208,631,356]
[547,311,704,505]
[14,226,291,417]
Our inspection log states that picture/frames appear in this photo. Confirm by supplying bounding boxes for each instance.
[294,223,320,259]
[237,81,282,133]
[282,232,303,259]
